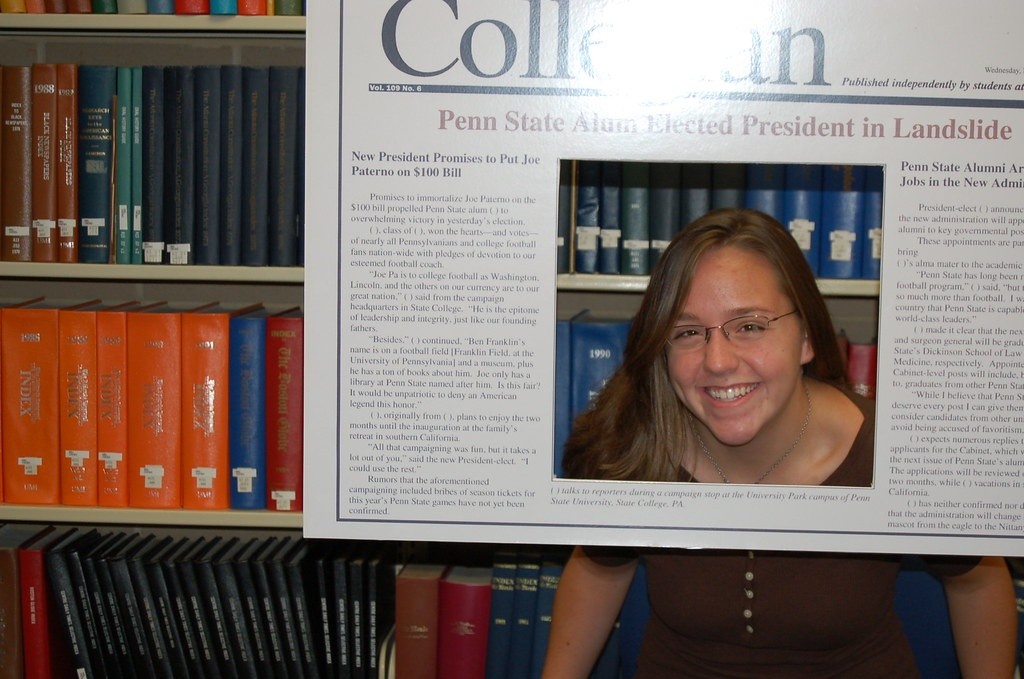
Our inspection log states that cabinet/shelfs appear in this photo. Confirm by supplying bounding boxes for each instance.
[0,15,884,679]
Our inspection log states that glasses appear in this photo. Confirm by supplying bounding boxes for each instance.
[664,310,798,350]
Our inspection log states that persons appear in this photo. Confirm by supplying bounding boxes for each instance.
[1006,557,1024,679]
[541,208,1017,678]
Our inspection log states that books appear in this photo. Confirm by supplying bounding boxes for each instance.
[0,64,305,267]
[394,542,651,679]
[0,0,307,15]
[833,332,877,400]
[551,308,639,479]
[0,524,398,679]
[556,160,884,282]
[0,296,304,513]
